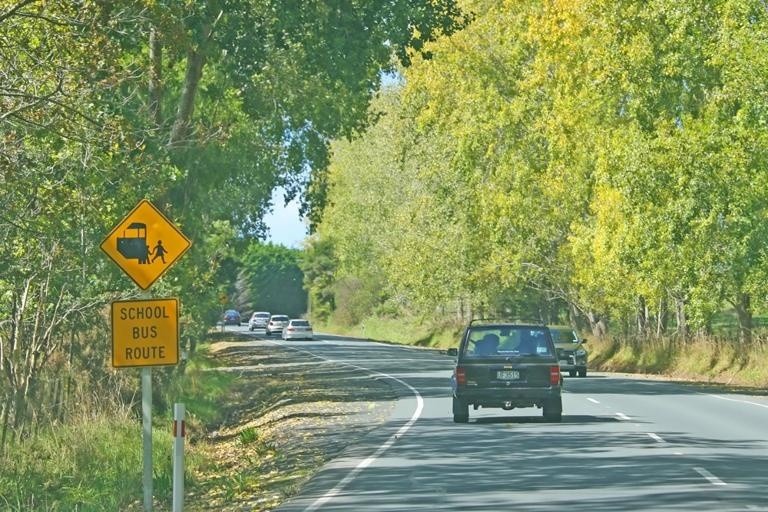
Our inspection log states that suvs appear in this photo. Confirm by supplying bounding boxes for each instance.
[446,317,563,422]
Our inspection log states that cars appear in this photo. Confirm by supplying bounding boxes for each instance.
[281,318,313,340]
[249,311,271,330]
[532,323,588,377]
[267,315,289,335]
[224,309,241,326]
